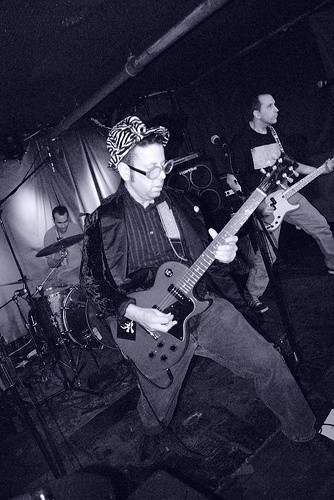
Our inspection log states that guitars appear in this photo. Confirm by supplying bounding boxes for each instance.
[256,156,334,231]
[110,151,299,370]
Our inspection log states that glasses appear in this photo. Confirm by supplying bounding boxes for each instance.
[128,160,175,180]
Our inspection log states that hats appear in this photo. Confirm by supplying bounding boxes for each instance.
[106,116,171,169]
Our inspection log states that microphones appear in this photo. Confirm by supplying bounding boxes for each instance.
[210,134,226,147]
[47,145,55,172]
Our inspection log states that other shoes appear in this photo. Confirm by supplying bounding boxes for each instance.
[286,431,334,465]
[243,296,268,313]
[140,430,170,460]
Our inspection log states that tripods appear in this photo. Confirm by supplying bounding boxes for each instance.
[1,248,104,412]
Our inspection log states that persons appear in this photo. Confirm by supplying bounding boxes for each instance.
[44,204,84,345]
[228,93,334,314]
[79,117,334,469]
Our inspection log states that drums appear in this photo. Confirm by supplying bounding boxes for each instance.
[32,284,73,338]
[60,283,120,351]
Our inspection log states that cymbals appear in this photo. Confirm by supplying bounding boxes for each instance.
[1,278,44,287]
[36,233,85,257]
